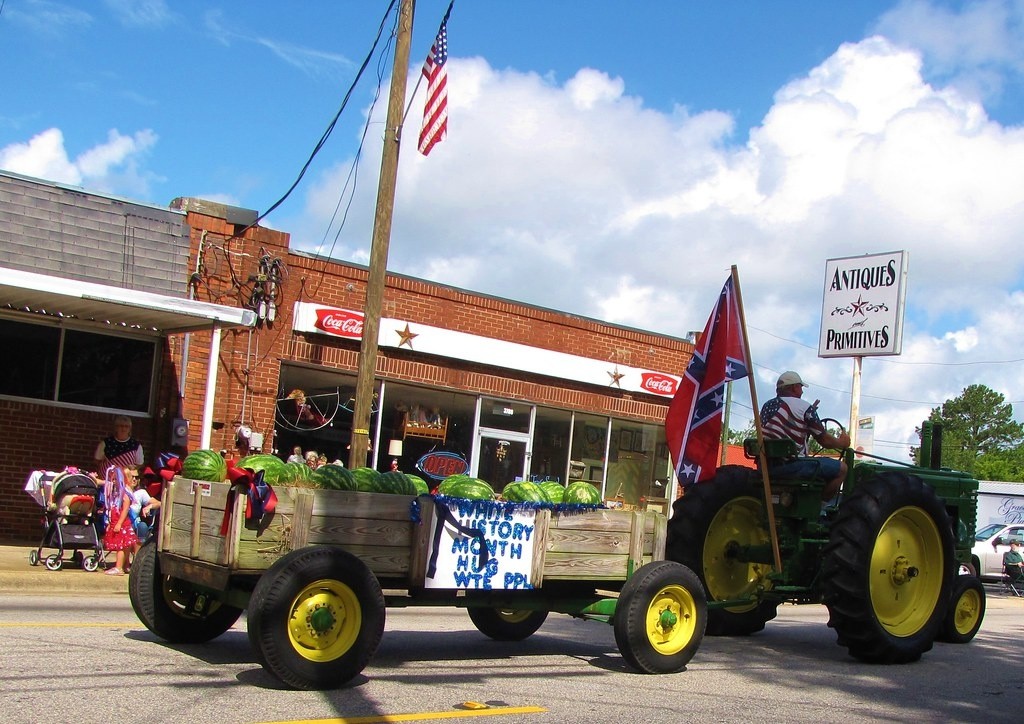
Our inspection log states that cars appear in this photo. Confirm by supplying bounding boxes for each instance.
[969,522,1024,581]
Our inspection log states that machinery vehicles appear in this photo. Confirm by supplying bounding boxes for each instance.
[663,416,988,669]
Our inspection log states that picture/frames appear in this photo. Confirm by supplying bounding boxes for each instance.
[590,465,608,492]
[619,428,633,452]
[633,431,650,455]
[605,497,625,509]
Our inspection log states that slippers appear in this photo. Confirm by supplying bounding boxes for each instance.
[105,565,131,576]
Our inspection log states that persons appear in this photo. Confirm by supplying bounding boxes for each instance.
[1003,539,1024,581]
[96,465,161,576]
[287,447,344,471]
[754,371,851,510]
[390,458,403,474]
[95,415,144,480]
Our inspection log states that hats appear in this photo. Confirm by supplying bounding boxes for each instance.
[776,371,809,389]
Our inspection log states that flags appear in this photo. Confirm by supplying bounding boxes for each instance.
[665,274,749,486]
[417,18,448,156]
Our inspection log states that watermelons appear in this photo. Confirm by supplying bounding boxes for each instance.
[182,448,604,506]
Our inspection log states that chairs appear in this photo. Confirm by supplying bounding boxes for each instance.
[98,486,158,569]
[999,551,1024,597]
[744,437,829,519]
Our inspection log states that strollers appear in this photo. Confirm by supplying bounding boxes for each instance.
[29,468,104,572]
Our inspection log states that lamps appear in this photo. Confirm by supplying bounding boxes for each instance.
[389,440,403,471]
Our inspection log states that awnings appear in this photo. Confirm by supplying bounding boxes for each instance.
[0,267,259,337]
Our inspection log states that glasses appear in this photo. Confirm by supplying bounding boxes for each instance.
[132,475,140,480]
[114,424,130,428]
